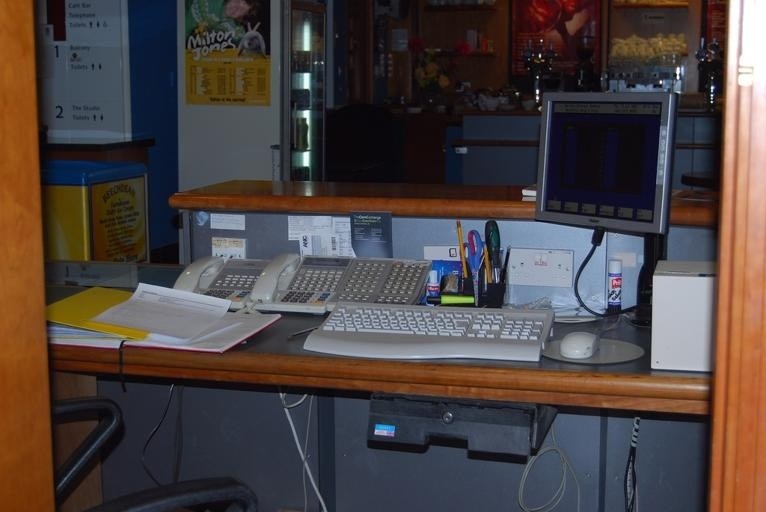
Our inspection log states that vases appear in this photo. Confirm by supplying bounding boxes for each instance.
[420,90,443,112]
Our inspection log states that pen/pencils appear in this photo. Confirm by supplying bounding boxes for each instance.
[455,218,469,278]
[289,326,318,337]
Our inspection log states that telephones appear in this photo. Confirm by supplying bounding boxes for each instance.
[173,253,354,315]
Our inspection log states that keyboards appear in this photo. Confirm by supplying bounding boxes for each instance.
[302,301,554,363]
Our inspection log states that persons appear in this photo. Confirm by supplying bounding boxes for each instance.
[185,0,261,48]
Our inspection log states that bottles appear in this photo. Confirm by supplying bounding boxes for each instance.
[295,118,308,151]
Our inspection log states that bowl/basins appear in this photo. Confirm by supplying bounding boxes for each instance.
[404,94,535,114]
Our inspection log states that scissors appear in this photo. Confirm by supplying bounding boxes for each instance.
[459,230,482,307]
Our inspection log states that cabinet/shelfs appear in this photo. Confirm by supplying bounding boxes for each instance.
[424,5,499,58]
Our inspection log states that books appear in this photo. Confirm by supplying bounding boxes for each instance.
[46,287,283,355]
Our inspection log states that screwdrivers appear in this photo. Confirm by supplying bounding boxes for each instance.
[485,221,500,284]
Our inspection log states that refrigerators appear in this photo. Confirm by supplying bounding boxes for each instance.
[174,0,323,264]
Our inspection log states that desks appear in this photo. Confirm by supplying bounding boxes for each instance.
[44,284,713,511]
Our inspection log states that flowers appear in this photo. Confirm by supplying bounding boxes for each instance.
[407,35,450,90]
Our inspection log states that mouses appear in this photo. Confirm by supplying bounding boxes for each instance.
[561,332,599,359]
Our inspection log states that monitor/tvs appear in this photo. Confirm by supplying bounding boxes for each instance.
[535,91,679,237]
